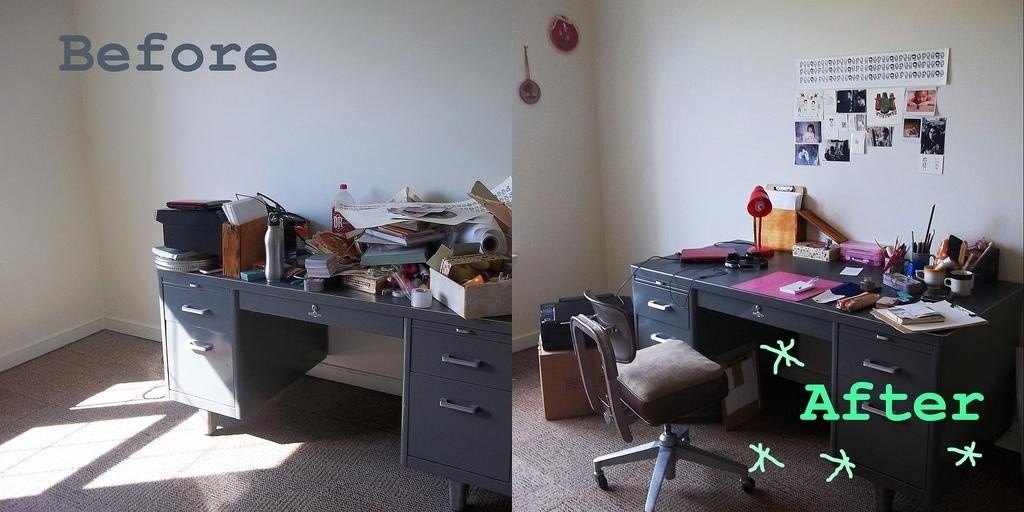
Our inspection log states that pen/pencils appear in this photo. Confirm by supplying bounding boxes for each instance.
[971,246,991,271]
[963,253,973,270]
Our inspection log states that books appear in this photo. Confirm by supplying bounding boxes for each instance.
[354,217,446,266]
[887,301,946,324]
[680,247,738,262]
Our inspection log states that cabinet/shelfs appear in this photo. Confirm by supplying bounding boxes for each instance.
[631,249,1023,512]
[157,269,512,511]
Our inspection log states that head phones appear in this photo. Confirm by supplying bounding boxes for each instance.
[724,251,769,271]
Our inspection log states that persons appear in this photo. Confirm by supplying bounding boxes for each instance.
[924,127,943,154]
[907,90,935,108]
[837,90,866,112]
[802,124,817,143]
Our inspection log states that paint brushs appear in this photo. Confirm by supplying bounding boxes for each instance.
[912,204,936,254]
[874,234,911,258]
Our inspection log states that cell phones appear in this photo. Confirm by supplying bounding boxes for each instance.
[198,263,222,275]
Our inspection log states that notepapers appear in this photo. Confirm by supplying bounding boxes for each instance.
[779,280,816,294]
[840,266,863,276]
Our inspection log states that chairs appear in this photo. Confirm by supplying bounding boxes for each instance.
[570,290,756,512]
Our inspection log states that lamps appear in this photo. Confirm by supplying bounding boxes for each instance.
[747,186,774,258]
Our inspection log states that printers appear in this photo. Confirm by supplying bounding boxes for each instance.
[537,294,632,352]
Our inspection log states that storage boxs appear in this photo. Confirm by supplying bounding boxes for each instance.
[538,333,607,419]
[429,266,512,319]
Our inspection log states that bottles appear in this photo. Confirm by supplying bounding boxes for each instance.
[331,183,357,235]
[263,209,286,285]
[859,276,876,293]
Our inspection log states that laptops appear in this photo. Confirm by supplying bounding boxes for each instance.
[680,248,736,264]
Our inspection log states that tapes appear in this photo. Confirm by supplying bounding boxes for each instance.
[411,288,432,308]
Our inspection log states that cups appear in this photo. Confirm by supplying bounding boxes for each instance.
[912,251,937,266]
[884,257,911,275]
[944,269,974,295]
[915,264,946,291]
